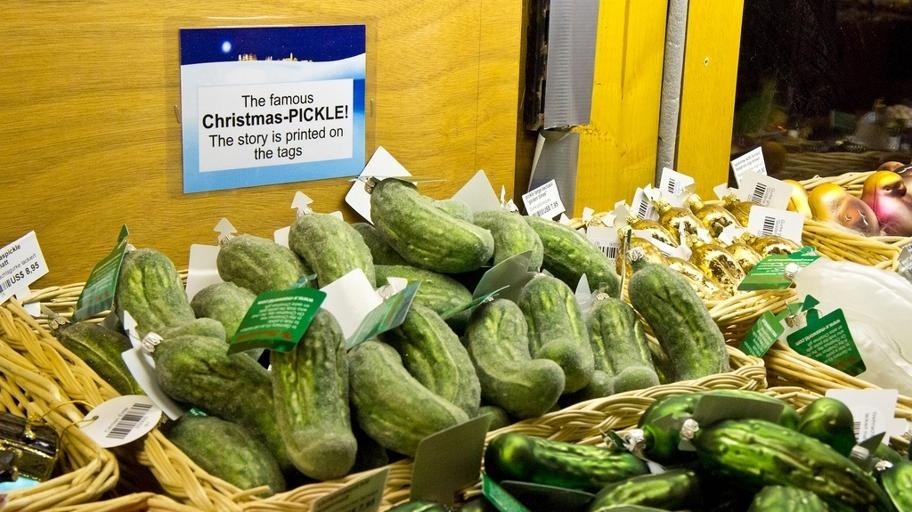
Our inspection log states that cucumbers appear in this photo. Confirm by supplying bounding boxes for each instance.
[482,389,912,512]
[59,170,730,494]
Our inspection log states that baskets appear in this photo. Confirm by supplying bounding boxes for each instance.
[736,107,912,179]
[56,491,202,512]
[761,336,912,422]
[18,266,771,511]
[565,198,903,345]
[347,385,912,511]
[0,338,120,512]
[800,169,912,245]
